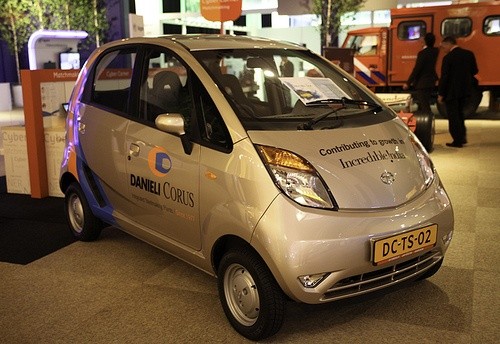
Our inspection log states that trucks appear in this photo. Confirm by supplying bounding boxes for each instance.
[304,0,500,112]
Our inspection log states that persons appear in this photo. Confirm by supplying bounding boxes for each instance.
[239,56,255,71]
[296,90,320,100]
[280,55,294,77]
[402,32,440,105]
[438,35,472,148]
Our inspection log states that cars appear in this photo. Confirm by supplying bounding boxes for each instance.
[59,32,454,340]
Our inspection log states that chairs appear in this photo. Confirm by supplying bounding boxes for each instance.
[139,71,184,121]
[198,74,255,143]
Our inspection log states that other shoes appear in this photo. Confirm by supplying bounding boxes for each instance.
[461,138,467,144]
[447,141,463,147]
